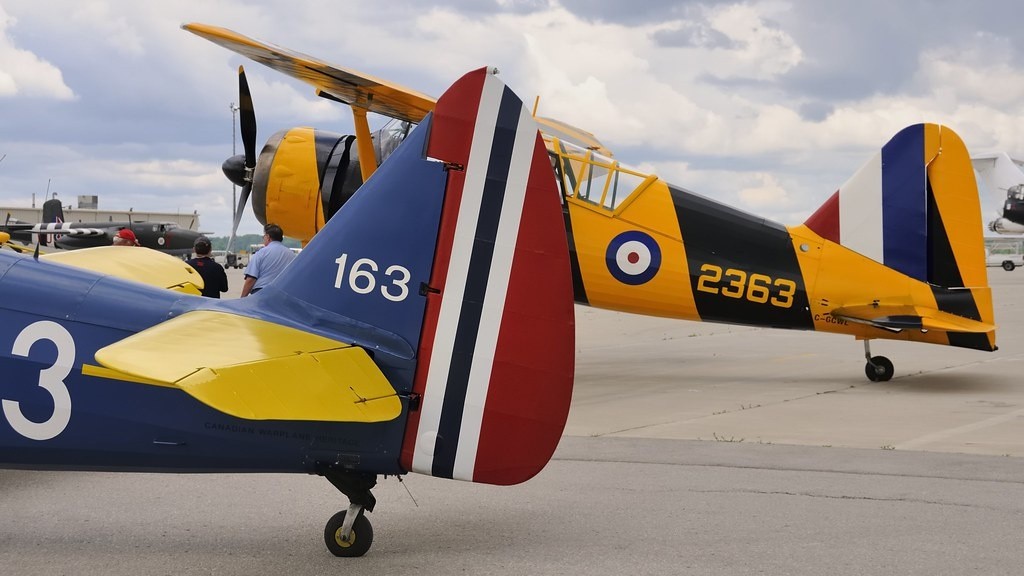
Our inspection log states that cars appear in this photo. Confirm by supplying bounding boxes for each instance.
[984,246,1024,271]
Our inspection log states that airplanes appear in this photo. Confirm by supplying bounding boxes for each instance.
[0,22,1024,560]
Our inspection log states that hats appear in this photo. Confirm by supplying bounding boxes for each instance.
[116,229,141,247]
[194,236,211,246]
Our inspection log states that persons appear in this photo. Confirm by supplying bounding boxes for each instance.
[111,228,140,247]
[241,223,298,298]
[185,250,191,261]
[183,236,229,298]
